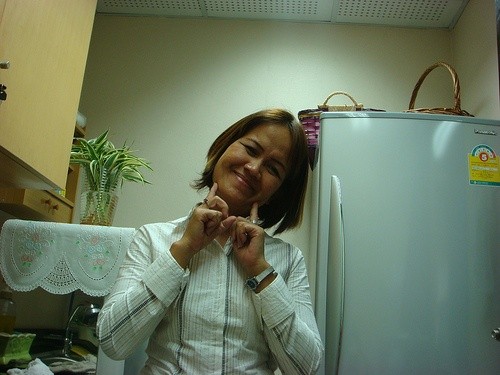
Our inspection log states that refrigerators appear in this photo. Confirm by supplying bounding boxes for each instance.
[255,110,500,375]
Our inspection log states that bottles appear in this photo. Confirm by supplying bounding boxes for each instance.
[0,289,16,334]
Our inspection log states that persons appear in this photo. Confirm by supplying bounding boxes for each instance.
[96,106,324,375]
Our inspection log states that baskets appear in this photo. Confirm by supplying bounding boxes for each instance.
[298,91,386,155]
[403,61,475,116]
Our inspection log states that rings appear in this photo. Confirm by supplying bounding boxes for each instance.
[204,197,209,209]
[249,219,258,224]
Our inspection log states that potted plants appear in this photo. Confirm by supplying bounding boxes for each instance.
[69,128,153,226]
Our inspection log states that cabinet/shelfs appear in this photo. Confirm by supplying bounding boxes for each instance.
[0,0,97,223]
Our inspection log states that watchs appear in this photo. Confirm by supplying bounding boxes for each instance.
[245,266,274,290]
